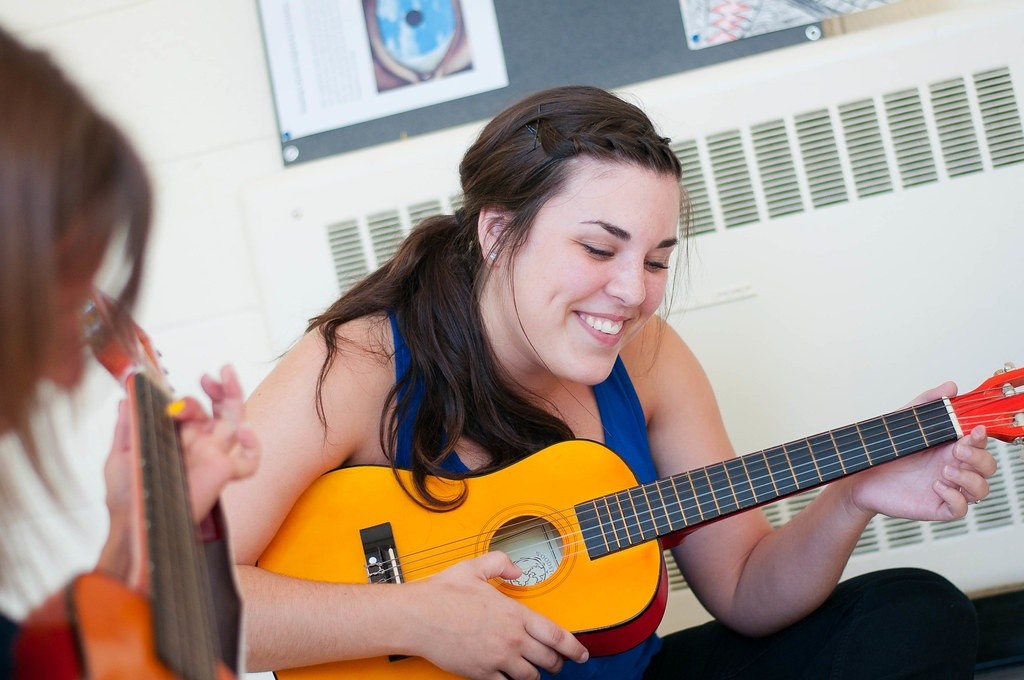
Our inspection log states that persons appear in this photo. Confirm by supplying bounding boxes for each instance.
[219,84,995,680]
[0,27,262,680]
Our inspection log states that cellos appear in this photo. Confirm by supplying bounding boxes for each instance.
[9,286,245,679]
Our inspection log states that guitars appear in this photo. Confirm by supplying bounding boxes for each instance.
[253,363,1024,680]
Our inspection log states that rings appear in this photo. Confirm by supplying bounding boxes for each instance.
[959,486,980,504]
